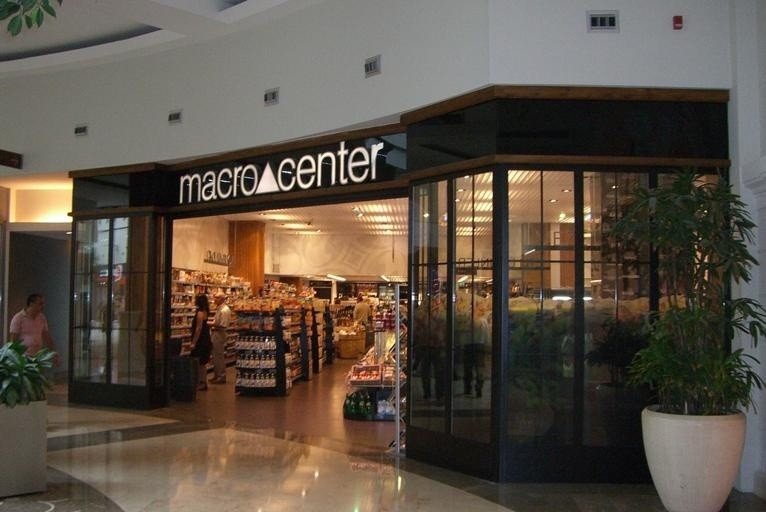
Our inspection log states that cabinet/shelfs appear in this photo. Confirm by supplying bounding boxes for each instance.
[170,272,407,423]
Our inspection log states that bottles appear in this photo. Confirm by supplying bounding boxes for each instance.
[236,336,277,388]
[344,391,374,416]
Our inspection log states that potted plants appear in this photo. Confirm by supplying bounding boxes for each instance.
[0,336,61,500]
[601,159,766,511]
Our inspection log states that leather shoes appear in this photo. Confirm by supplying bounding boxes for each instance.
[208,377,227,384]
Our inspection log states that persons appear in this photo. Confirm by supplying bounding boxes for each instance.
[189,293,213,392]
[10,293,59,366]
[419,299,447,404]
[462,307,489,403]
[207,291,231,385]
[353,290,370,327]
